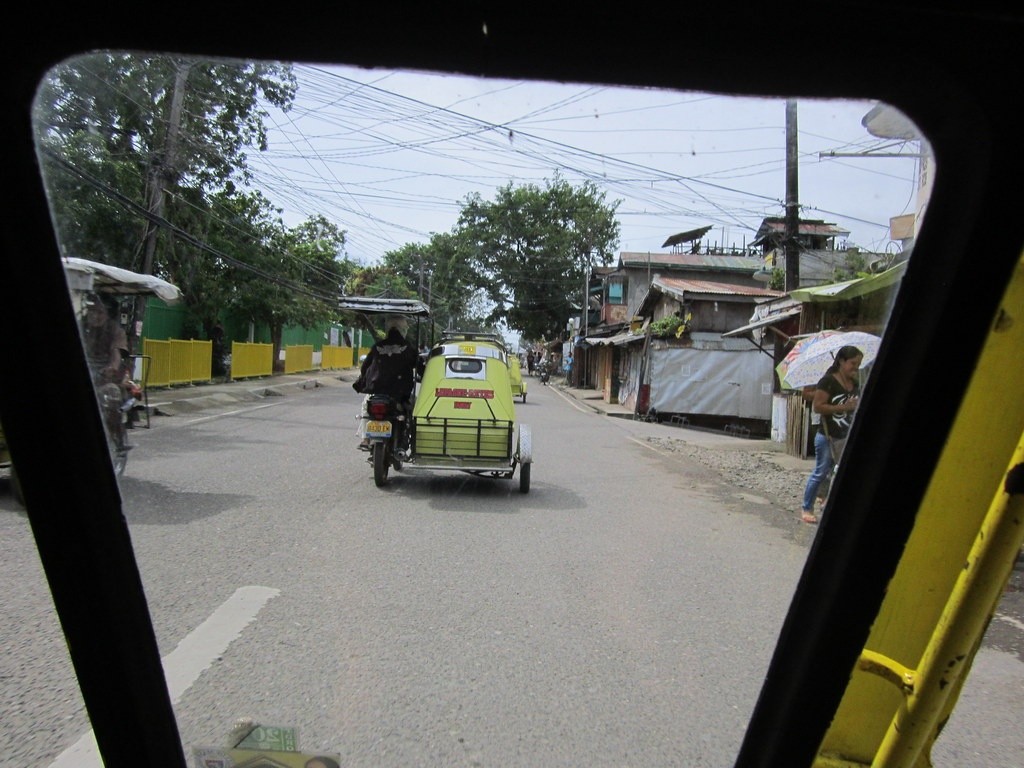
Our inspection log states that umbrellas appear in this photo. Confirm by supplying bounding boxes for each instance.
[775,330,883,400]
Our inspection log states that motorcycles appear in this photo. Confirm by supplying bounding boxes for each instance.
[536,360,550,386]
[337,295,532,495]
[0,255,183,509]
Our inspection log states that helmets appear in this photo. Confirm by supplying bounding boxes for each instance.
[388,316,409,339]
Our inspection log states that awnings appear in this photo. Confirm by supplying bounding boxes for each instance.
[662,225,713,248]
[585,330,646,345]
[790,260,908,303]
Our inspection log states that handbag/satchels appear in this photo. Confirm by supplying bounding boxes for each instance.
[830,437,847,462]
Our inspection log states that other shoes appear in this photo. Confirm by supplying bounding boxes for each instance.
[357,444,369,451]
[800,508,816,523]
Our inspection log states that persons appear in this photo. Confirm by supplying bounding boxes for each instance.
[353,315,429,451]
[799,344,864,523]
[564,352,573,388]
[77,291,133,451]
[527,351,542,377]
